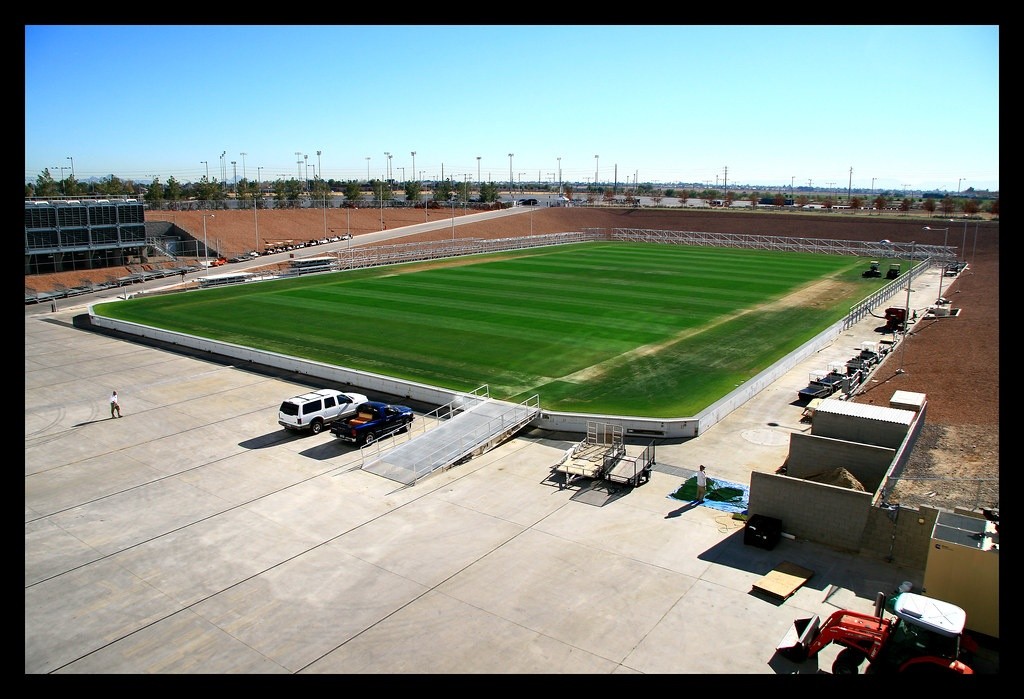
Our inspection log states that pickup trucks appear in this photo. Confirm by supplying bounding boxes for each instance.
[328,400,416,447]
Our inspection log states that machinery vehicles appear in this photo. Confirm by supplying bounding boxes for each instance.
[774,580,974,674]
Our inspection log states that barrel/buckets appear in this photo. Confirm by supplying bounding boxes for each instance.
[903,582,913,592]
[899,585,910,592]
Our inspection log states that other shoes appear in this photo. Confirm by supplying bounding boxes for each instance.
[699,500,705,504]
[112,416,116,419]
[695,497,699,501]
[118,415,123,418]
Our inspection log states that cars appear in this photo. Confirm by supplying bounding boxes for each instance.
[522,198,541,205]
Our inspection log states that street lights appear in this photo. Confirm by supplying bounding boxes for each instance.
[457,173,472,215]
[878,238,916,374]
[595,154,599,198]
[557,157,561,194]
[199,149,425,195]
[530,200,541,246]
[871,177,877,205]
[508,154,513,192]
[450,201,455,250]
[67,156,75,184]
[424,181,432,223]
[203,214,215,277]
[518,172,526,194]
[964,213,980,263]
[957,178,966,211]
[477,156,481,186]
[808,178,811,211]
[921,226,950,322]
[950,218,967,263]
[792,176,795,207]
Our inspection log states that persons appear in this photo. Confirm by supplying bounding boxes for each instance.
[110,390,123,418]
[180,271,186,283]
[696,465,707,503]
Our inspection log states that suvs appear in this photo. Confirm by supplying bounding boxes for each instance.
[277,388,369,435]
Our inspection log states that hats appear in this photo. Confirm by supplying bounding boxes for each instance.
[700,465,705,469]
